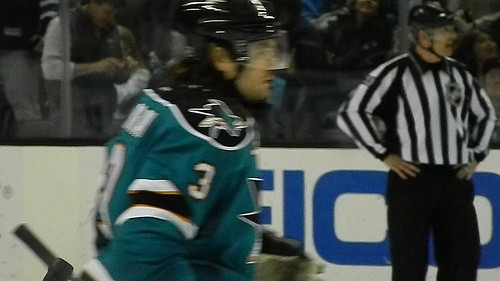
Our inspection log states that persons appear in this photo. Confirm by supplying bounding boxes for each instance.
[0,0,500,281]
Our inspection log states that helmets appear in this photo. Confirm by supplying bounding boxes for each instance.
[180,0,281,43]
[407,4,456,30]
